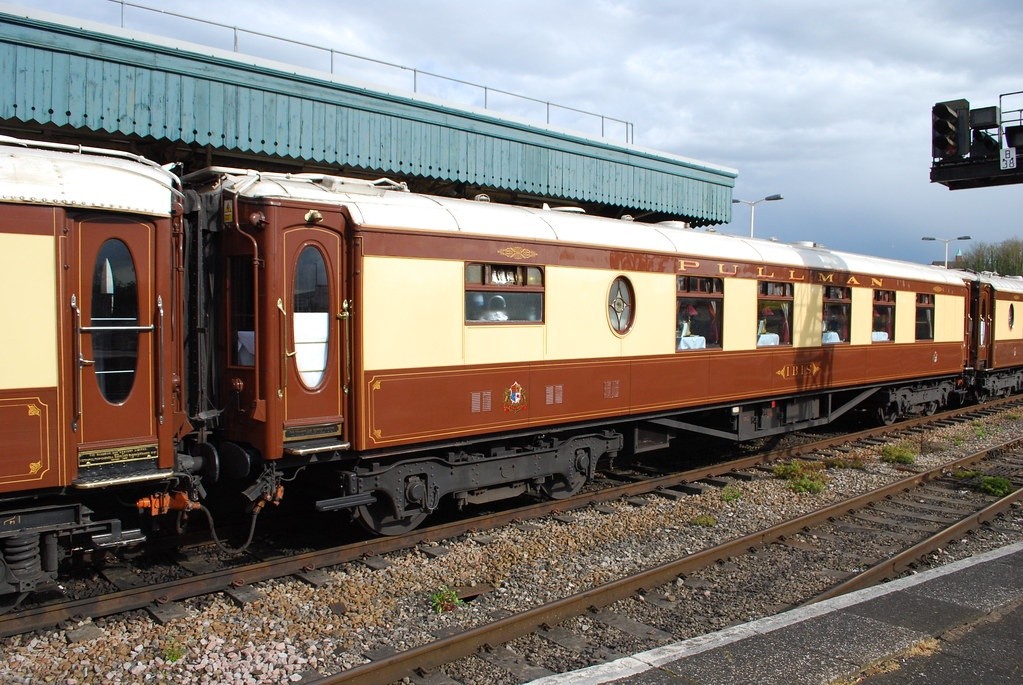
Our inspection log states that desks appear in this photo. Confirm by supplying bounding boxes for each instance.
[676,334,706,350]
[822,331,840,344]
[757,332,780,347]
[872,331,889,342]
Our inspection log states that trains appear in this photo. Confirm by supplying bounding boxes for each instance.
[0,131,1023,612]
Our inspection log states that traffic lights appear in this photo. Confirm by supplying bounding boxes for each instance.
[930,98,970,159]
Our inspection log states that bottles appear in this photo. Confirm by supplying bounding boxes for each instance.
[491,269,516,285]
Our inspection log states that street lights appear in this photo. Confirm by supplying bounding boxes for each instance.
[732,194,785,238]
[922,235,972,270]
